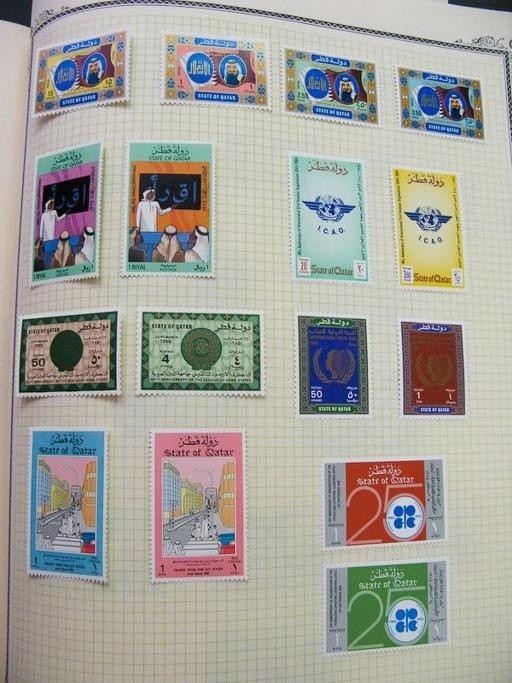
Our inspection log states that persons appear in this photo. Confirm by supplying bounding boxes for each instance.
[86,58,104,84]
[34,199,94,272]
[224,60,244,84]
[130,186,209,263]
[448,94,465,119]
[337,78,357,101]
